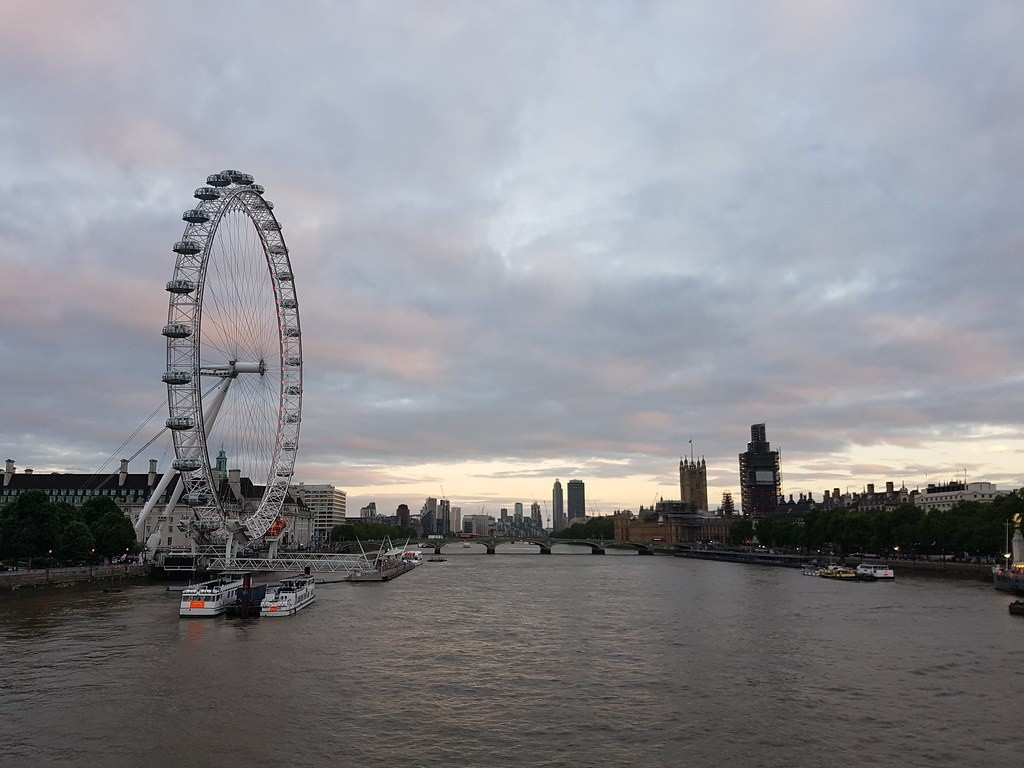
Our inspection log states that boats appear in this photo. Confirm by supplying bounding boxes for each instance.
[993,518,1024,596]
[1009,600,1024,615]
[801,560,896,581]
[257,573,318,618]
[463,543,471,548]
[179,575,254,618]
[399,550,424,567]
